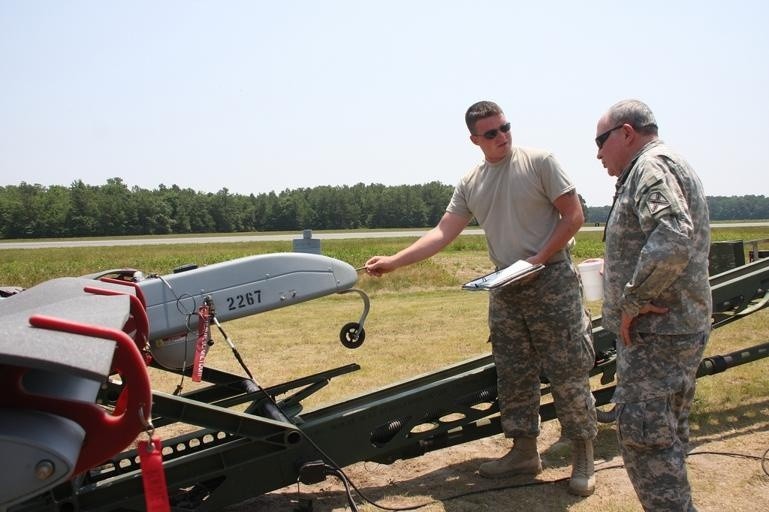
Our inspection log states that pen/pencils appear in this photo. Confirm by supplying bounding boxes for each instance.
[355,266,367,271]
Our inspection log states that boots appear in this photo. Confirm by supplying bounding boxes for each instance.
[478,434,543,478]
[568,440,595,498]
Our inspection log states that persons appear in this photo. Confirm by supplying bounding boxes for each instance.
[597,100,713,512]
[365,101,596,496]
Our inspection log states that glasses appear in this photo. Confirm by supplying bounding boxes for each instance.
[473,122,511,140]
[595,125,622,150]
